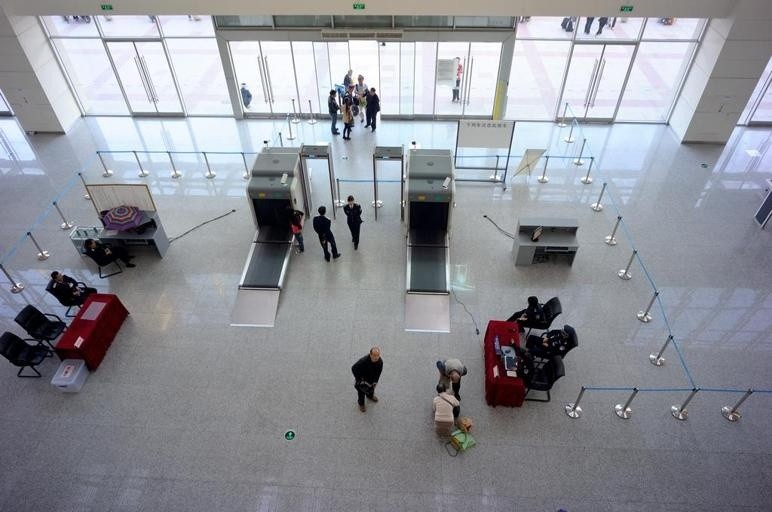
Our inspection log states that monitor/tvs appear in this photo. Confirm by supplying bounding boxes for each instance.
[531,224,544,242]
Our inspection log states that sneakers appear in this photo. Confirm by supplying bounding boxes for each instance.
[126,263,135,268]
[360,405,366,412]
[372,396,378,402]
[333,119,375,140]
[297,237,358,262]
[130,255,136,258]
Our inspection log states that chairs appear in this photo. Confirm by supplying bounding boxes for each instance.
[526,296,562,339]
[523,356,565,402]
[14,304,69,352]
[530,325,579,364]
[81,243,123,279]
[0,331,53,379]
[45,279,86,317]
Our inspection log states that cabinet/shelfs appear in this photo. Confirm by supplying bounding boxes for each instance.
[511,217,579,268]
[70,226,102,257]
[98,211,169,259]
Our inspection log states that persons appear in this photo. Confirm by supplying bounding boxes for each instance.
[313,206,340,261]
[510,338,534,383]
[506,296,544,332]
[240,83,252,108]
[341,97,353,139]
[432,383,460,434]
[344,70,353,85]
[328,90,340,134]
[343,196,362,249]
[518,15,677,35]
[63,15,202,25]
[364,88,380,132]
[452,57,462,102]
[355,75,369,122]
[352,348,383,412]
[85,239,136,267]
[345,86,354,132]
[51,271,97,301]
[290,215,304,254]
[437,359,467,401]
[525,329,570,358]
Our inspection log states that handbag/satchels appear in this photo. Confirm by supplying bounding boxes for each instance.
[458,417,473,432]
[354,96,359,106]
[449,429,475,450]
[354,381,376,395]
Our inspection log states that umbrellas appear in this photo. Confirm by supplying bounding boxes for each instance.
[103,206,143,231]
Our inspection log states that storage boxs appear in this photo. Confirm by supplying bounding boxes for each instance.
[50,358,90,393]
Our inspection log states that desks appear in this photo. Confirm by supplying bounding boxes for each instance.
[54,293,130,372]
[484,319,522,409]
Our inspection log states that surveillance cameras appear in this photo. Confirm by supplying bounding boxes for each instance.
[411,140,417,146]
[442,176,452,190]
[280,172,288,185]
[263,139,269,145]
